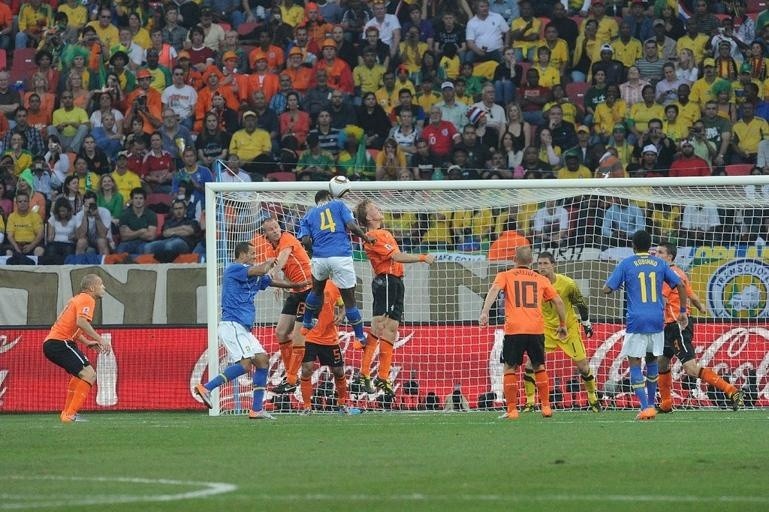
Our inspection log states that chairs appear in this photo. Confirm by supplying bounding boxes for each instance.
[11,21,593,237]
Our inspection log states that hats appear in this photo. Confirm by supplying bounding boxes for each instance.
[59,0,753,169]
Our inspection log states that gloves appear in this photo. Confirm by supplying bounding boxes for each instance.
[582,320,595,339]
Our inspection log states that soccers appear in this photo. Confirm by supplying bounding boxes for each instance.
[329,175,352,197]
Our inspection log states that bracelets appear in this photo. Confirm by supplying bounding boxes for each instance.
[560,320,566,327]
[680,306,686,312]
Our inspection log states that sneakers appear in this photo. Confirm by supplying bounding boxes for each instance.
[339,403,349,415]
[523,403,535,412]
[374,378,394,396]
[657,406,674,414]
[301,408,313,415]
[193,382,213,409]
[591,400,601,413]
[541,405,553,418]
[498,412,520,420]
[359,373,376,394]
[730,388,745,412]
[248,409,274,419]
[636,407,656,420]
[60,412,90,423]
[272,377,297,394]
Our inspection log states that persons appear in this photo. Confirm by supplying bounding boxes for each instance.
[261,218,313,394]
[522,252,602,414]
[655,243,746,414]
[296,190,378,350]
[193,242,312,420]
[354,200,435,398]
[300,278,351,417]
[42,274,112,422]
[603,229,689,421]
[478,246,569,419]
